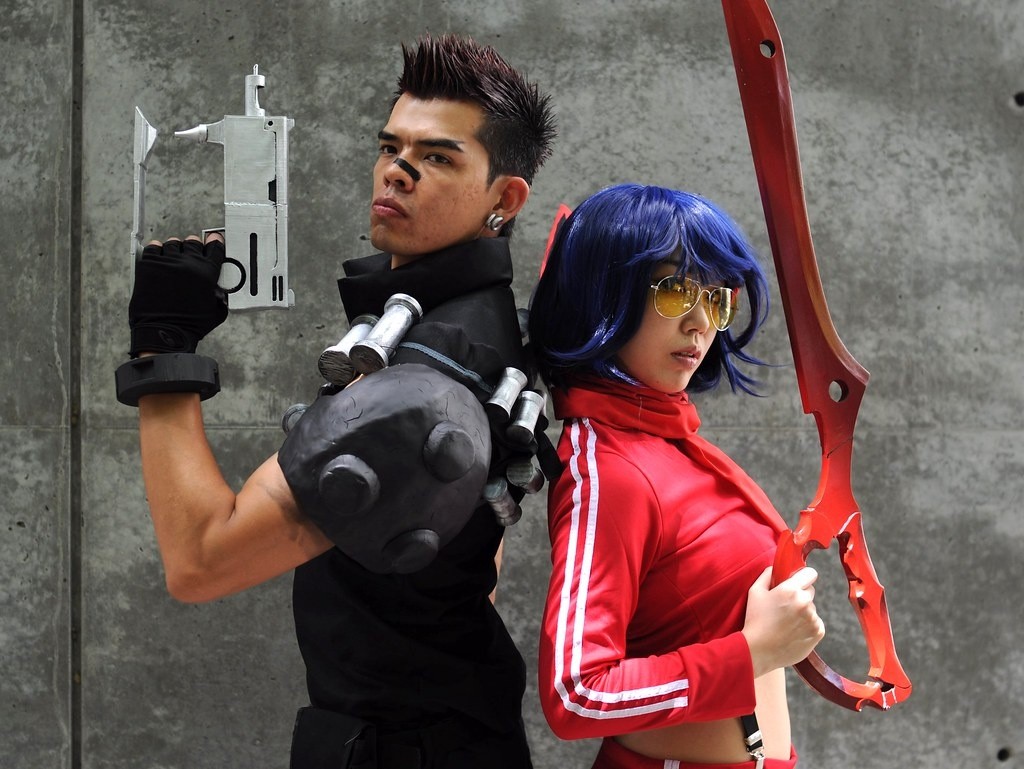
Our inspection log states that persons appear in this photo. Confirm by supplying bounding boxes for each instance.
[527,186,825,769]
[114,32,560,769]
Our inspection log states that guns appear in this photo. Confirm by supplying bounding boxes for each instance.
[128,63,296,310]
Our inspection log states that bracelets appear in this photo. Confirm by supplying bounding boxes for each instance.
[115,354,221,408]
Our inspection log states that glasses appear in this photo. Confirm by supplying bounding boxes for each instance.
[649,274,740,330]
